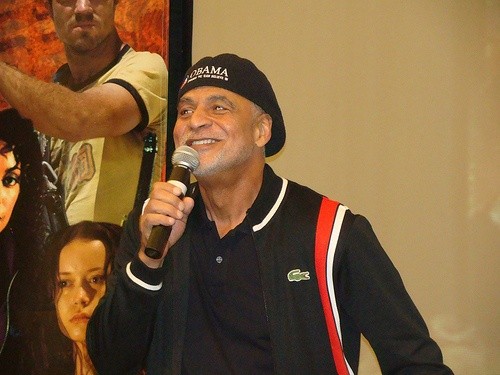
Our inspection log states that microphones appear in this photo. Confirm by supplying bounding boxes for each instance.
[144,146,200,260]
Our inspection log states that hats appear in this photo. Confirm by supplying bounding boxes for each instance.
[178,52,287,157]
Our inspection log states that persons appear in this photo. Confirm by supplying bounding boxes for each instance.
[86,54,454,375]
[0,108,68,375]
[33,221,122,375]
[0,1,168,226]
